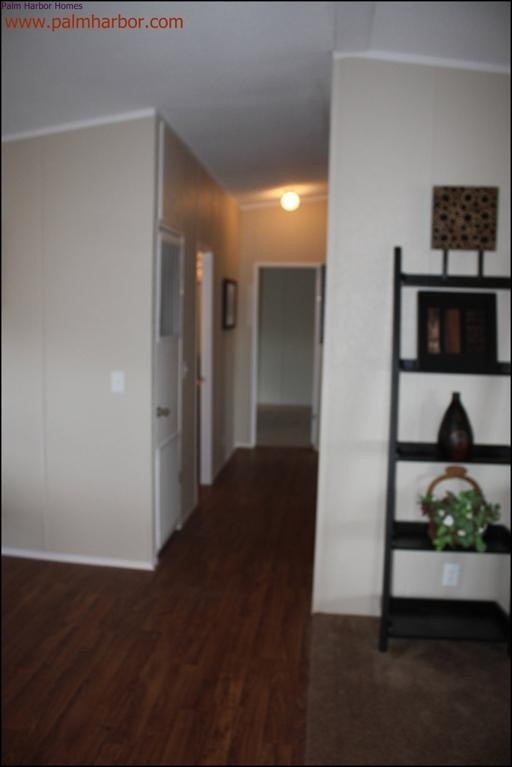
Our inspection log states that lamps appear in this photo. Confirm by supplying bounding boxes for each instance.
[280,191,300,213]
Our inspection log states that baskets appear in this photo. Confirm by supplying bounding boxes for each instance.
[420,463,492,547]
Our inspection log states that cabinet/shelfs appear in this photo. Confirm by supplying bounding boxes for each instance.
[379,247,512,655]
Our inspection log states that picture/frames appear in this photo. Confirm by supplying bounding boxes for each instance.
[222,276,237,328]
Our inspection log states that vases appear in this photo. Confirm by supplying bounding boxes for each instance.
[437,391,474,459]
